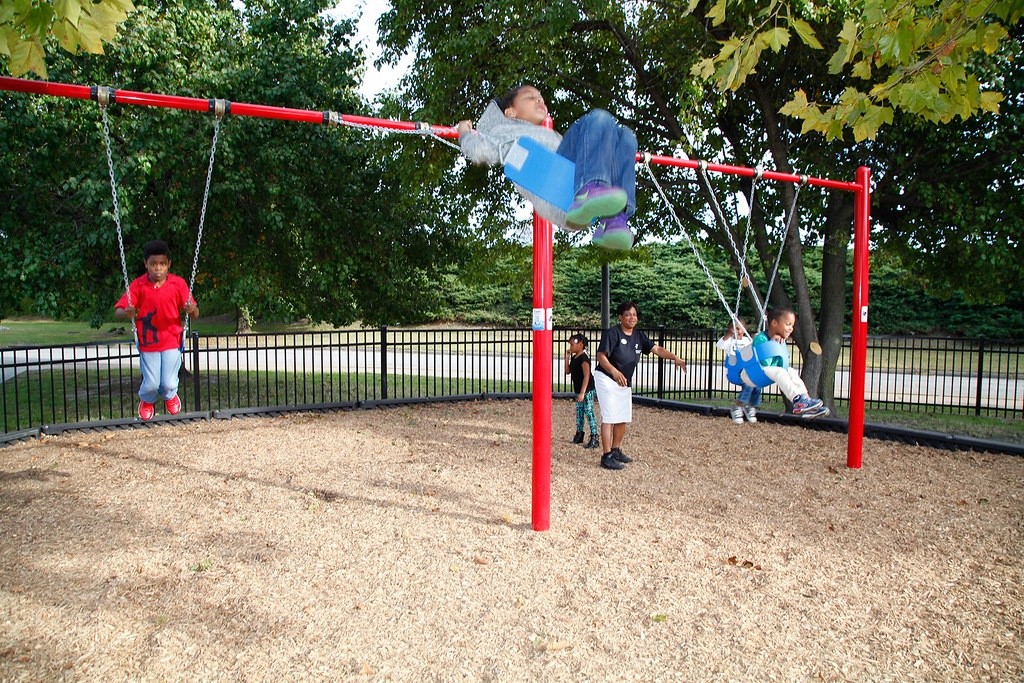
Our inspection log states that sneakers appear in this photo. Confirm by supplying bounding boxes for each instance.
[138,400,154,421]
[592,212,634,250]
[600,448,634,470]
[164,394,181,415]
[566,182,628,226]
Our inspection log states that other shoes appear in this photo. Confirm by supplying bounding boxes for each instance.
[570,431,585,444]
[743,405,757,423]
[730,404,744,424]
[585,433,600,449]
[792,397,830,419]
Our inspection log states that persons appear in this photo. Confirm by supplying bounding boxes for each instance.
[717,315,760,424]
[592,300,687,470]
[564,332,601,450]
[456,84,637,250]
[740,303,830,419]
[114,240,200,420]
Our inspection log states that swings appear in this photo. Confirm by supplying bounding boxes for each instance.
[94,93,223,362]
[329,114,601,231]
[693,158,809,387]
[640,151,790,389]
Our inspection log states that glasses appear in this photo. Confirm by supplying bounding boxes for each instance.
[568,337,582,344]
